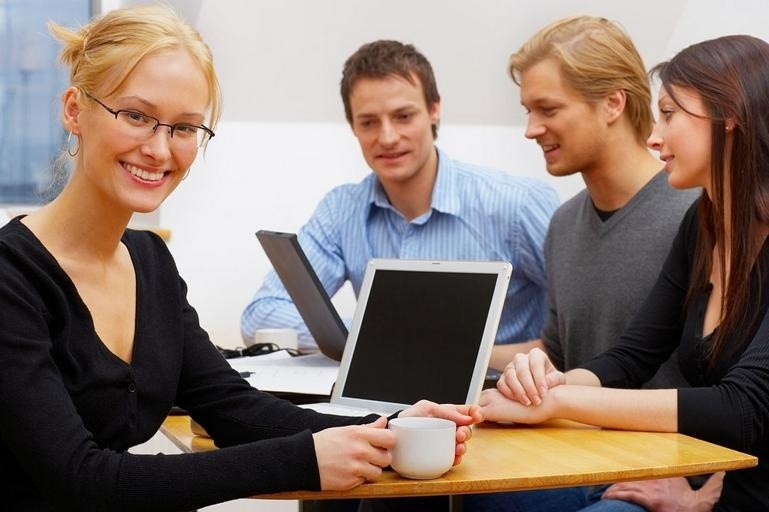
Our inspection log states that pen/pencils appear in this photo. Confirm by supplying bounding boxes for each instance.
[239,371,256,378]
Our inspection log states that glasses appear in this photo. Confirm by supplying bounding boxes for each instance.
[80,88,214,151]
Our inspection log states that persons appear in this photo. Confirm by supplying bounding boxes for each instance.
[0,3,487,511]
[479,33,768,511]
[468,13,726,511]
[238,37,562,383]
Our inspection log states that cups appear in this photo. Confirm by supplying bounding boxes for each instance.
[386,417,456,481]
[253,327,298,357]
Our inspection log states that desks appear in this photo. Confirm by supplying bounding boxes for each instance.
[155,338,760,500]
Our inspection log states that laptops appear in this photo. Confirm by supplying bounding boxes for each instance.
[254,229,504,387]
[191,258,513,439]
